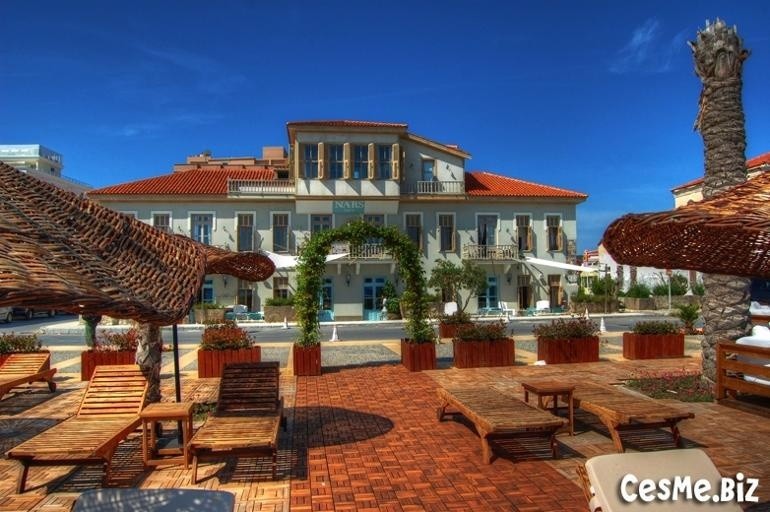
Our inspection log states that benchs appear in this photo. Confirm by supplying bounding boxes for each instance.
[715,337,770,400]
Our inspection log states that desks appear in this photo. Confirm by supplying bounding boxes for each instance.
[521,381,576,437]
[139,401,196,472]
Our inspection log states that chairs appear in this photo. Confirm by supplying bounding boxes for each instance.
[1,351,57,401]
[4,363,157,494]
[187,361,285,485]
[498,301,516,316]
[444,302,458,315]
[543,381,695,454]
[70,486,235,512]
[576,447,746,512]
[534,300,550,316]
[436,385,570,466]
